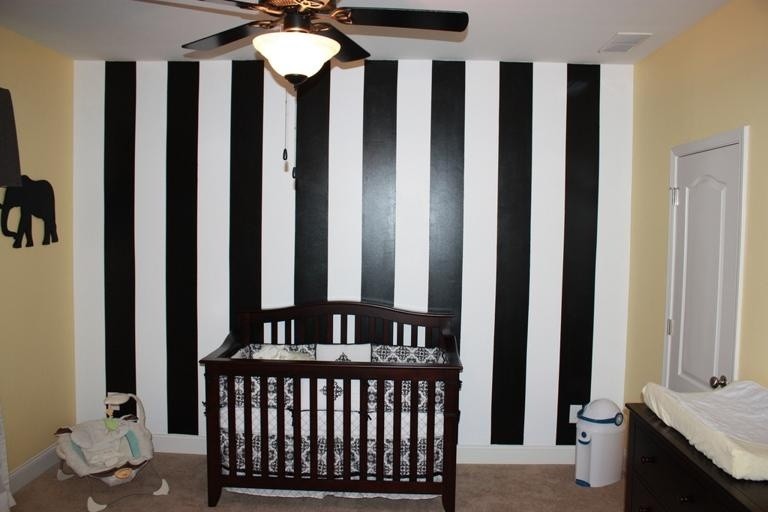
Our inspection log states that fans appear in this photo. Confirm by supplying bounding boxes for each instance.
[181,1,469,65]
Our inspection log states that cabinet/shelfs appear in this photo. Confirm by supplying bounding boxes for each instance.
[623,403,768,512]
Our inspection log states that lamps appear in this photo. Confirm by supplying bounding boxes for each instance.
[252,7,343,89]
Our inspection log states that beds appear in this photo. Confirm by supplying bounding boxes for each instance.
[198,298,464,511]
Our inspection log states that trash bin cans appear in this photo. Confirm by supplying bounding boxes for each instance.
[575,398,625,488]
